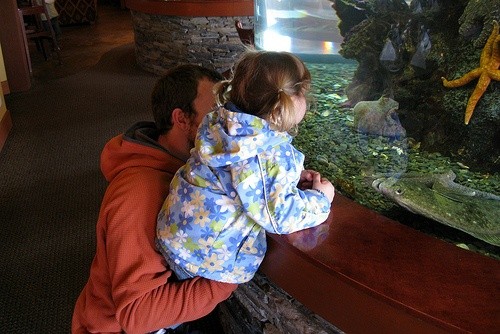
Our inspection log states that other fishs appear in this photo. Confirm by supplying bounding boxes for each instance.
[370,177,500,249]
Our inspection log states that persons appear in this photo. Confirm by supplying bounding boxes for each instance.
[151,48,336,334]
[68,63,242,333]
